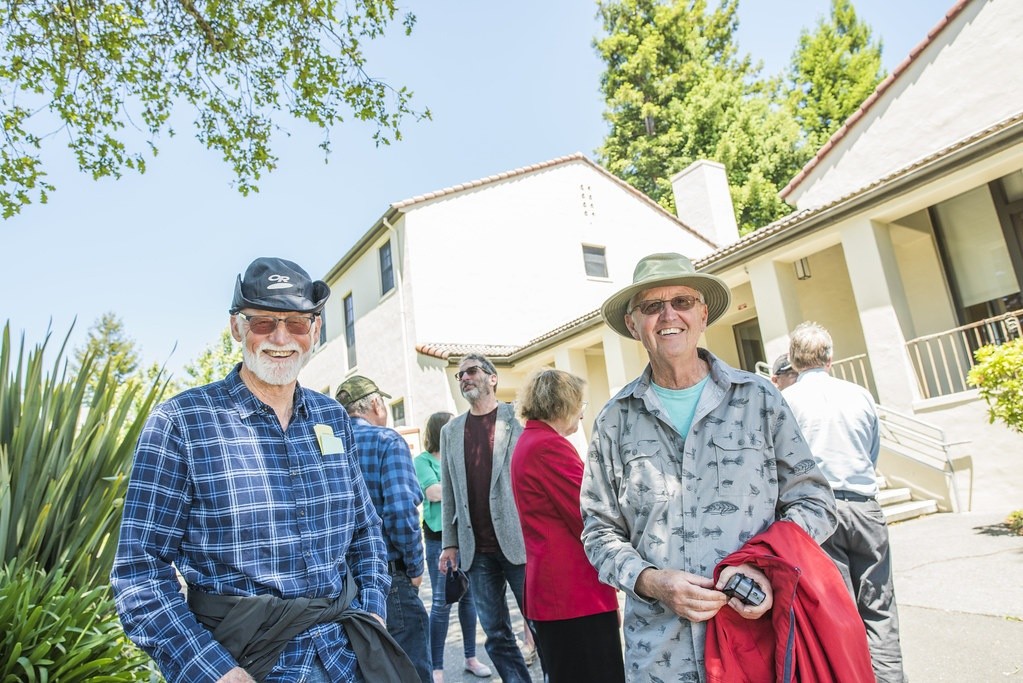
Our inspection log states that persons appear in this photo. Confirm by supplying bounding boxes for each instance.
[337,376,433,683]
[780,322,904,683]
[772,353,797,391]
[413,412,492,683]
[580,252,838,683]
[111,257,424,683]
[510,369,626,683]
[440,353,551,683]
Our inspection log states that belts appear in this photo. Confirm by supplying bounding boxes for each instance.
[387,560,405,574]
[832,490,876,502]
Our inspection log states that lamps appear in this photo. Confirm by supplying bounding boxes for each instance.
[794,256,812,281]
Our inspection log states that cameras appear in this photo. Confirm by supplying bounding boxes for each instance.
[724,572,766,606]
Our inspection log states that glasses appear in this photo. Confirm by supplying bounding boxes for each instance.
[628,295,704,316]
[455,366,493,381]
[236,310,321,335]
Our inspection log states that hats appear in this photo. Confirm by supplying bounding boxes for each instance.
[601,252,731,339]
[445,566,469,604]
[334,375,393,407]
[772,354,793,375]
[229,256,331,316]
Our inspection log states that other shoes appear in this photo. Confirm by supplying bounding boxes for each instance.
[433,669,443,683]
[520,644,537,666]
[464,657,491,676]
